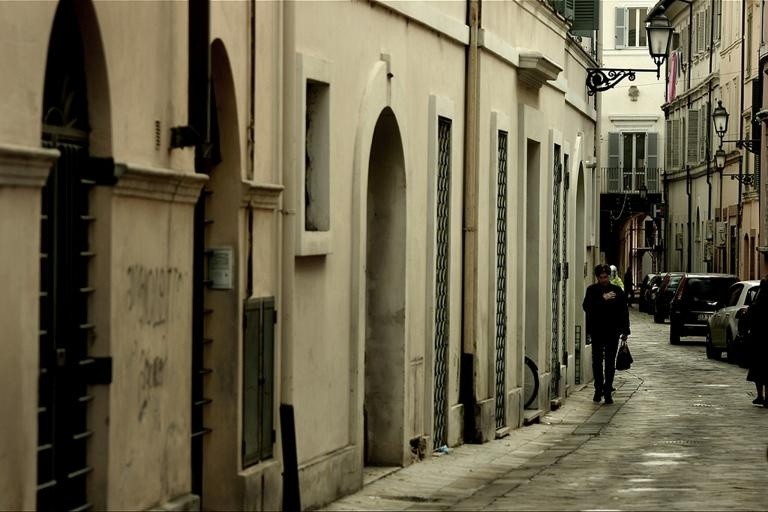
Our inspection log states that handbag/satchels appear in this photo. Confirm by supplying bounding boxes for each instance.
[616,342,633,370]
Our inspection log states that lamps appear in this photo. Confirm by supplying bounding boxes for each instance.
[638,182,669,209]
[585,3,676,97]
[711,99,762,147]
[714,145,761,192]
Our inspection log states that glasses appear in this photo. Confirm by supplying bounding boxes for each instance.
[598,275,608,278]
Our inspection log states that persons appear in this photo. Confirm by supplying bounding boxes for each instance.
[741,277,768,407]
[623,267,636,309]
[608,264,624,292]
[581,264,631,403]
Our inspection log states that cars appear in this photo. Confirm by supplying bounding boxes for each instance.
[637,272,761,364]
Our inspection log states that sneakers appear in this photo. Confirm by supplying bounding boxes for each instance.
[593,388,602,402]
[627,304,633,308]
[602,390,614,404]
[751,395,767,407]
